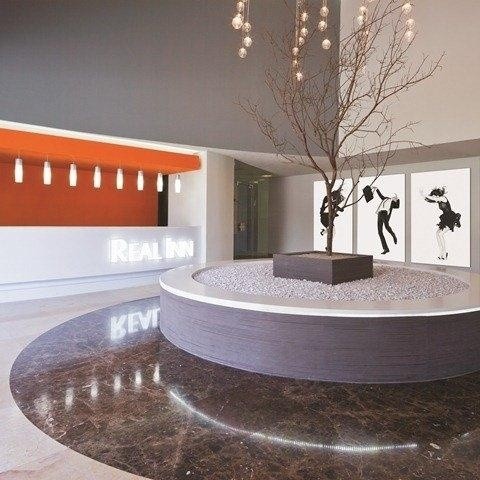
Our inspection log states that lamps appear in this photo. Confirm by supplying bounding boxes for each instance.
[43,156,52,185]
[137,166,144,191]
[116,164,124,190]
[156,169,164,192]
[175,173,182,193]
[93,162,102,188]
[14,152,24,183]
[69,161,78,186]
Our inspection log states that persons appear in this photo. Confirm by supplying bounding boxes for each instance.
[371,186,400,254]
[419,183,462,262]
[320,188,344,252]
